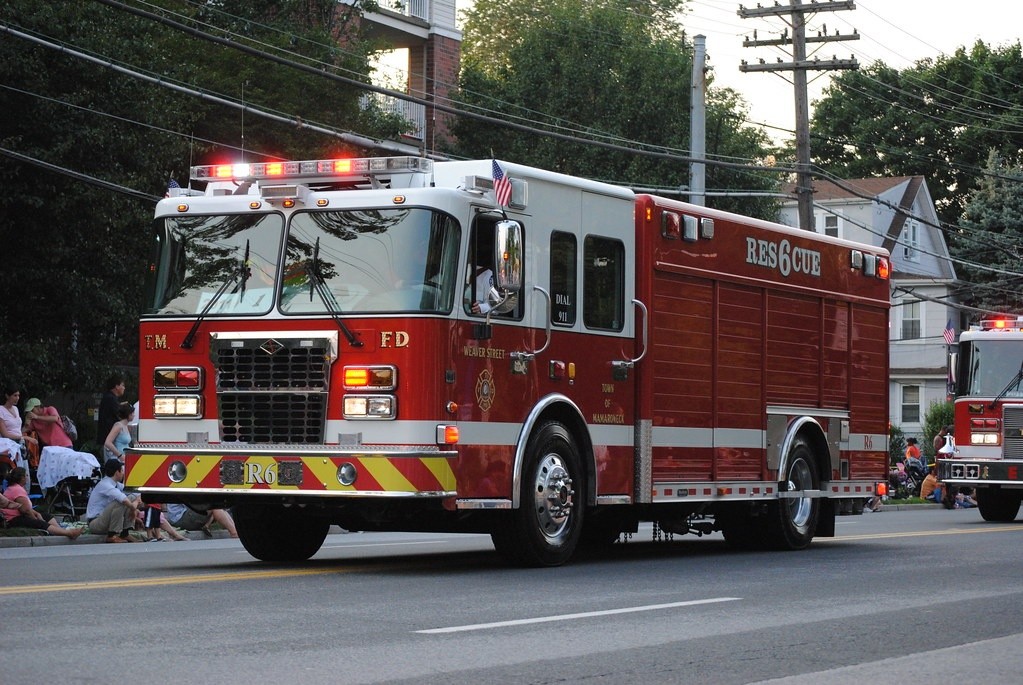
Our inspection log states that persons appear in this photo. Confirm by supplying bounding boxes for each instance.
[0,494,23,509]
[414,243,500,315]
[921,426,977,508]
[866,496,883,512]
[25,399,82,496]
[86,377,238,542]
[0,387,38,466]
[905,437,927,490]
[0,467,85,539]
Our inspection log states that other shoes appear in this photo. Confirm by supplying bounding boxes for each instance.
[201,526,214,537]
[148,536,157,542]
[106,534,128,543]
[173,535,192,541]
[158,536,168,542]
[72,526,84,540]
[120,535,137,542]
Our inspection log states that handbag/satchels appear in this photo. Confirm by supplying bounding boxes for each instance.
[61,416,77,441]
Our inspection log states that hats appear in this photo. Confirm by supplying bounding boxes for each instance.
[25,397,41,412]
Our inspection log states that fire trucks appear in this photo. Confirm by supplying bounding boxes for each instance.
[933,318,1023,523]
[119,154,892,570]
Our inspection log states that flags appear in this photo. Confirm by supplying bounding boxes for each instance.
[492,159,512,208]
[165,179,180,198]
[944,318,955,346]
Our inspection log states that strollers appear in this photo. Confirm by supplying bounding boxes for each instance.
[903,458,925,496]
[37,446,103,525]
